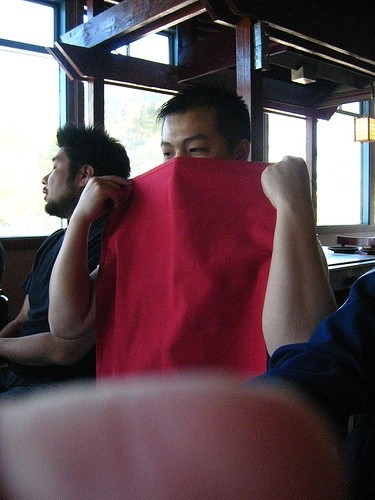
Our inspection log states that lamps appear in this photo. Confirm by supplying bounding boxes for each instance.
[353,115,375,143]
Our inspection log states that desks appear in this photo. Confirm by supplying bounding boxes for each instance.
[322,228,375,292]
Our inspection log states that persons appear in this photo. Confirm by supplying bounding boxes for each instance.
[0,122,131,401]
[48,82,338,388]
[244,265,375,500]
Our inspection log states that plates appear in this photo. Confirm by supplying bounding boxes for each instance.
[328,247,358,252]
